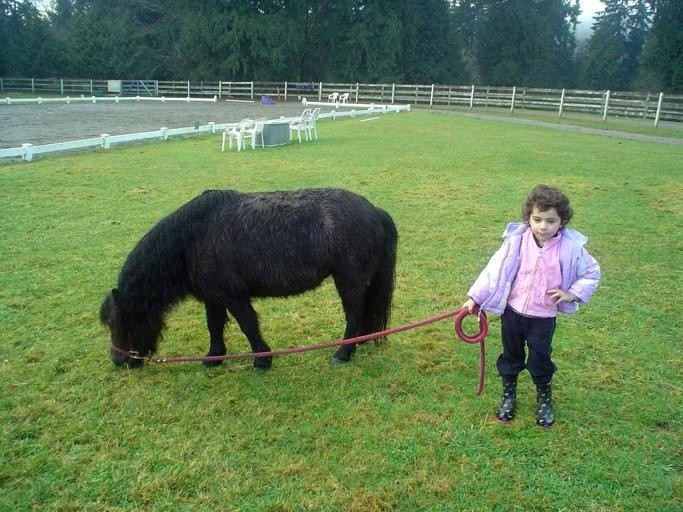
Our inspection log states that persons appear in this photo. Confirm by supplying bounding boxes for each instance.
[461,183,601,427]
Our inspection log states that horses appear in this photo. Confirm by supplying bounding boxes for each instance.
[98,187,398,371]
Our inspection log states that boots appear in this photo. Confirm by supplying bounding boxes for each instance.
[498,375,517,420]
[535,383,554,425]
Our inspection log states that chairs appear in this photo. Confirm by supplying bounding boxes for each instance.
[217,93,353,152]
